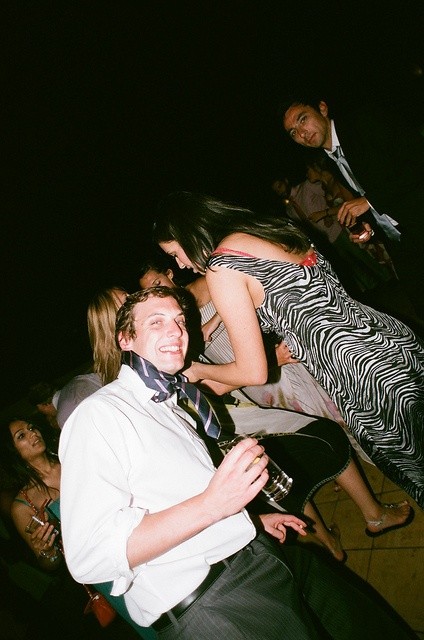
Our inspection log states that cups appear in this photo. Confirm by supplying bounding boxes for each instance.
[24,514,70,552]
[341,218,375,243]
[224,432,294,502]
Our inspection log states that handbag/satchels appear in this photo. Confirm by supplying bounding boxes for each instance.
[18,488,118,630]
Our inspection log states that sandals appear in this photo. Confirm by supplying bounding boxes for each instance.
[327,524,348,565]
[364,501,416,538]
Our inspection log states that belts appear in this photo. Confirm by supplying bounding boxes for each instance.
[151,551,239,633]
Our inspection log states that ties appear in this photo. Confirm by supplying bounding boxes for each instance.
[120,349,223,440]
[330,153,390,243]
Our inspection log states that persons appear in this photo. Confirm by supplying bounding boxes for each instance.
[27,375,104,431]
[56,287,420,640]
[134,262,377,464]
[151,191,424,512]
[3,414,61,568]
[272,93,423,285]
[85,288,414,564]
